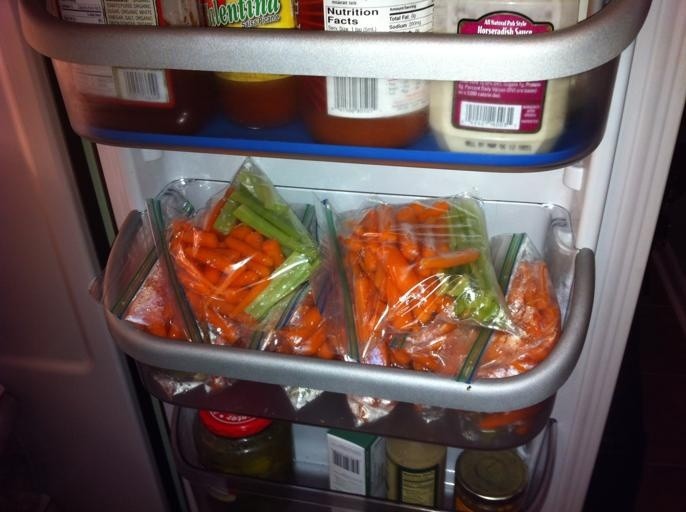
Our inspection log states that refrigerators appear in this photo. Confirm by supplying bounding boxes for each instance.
[0,1,686,512]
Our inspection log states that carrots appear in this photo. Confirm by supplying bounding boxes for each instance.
[121,181,562,440]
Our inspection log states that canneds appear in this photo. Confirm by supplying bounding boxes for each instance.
[191,411,294,511]
[454,446,531,510]
[384,438,447,511]
